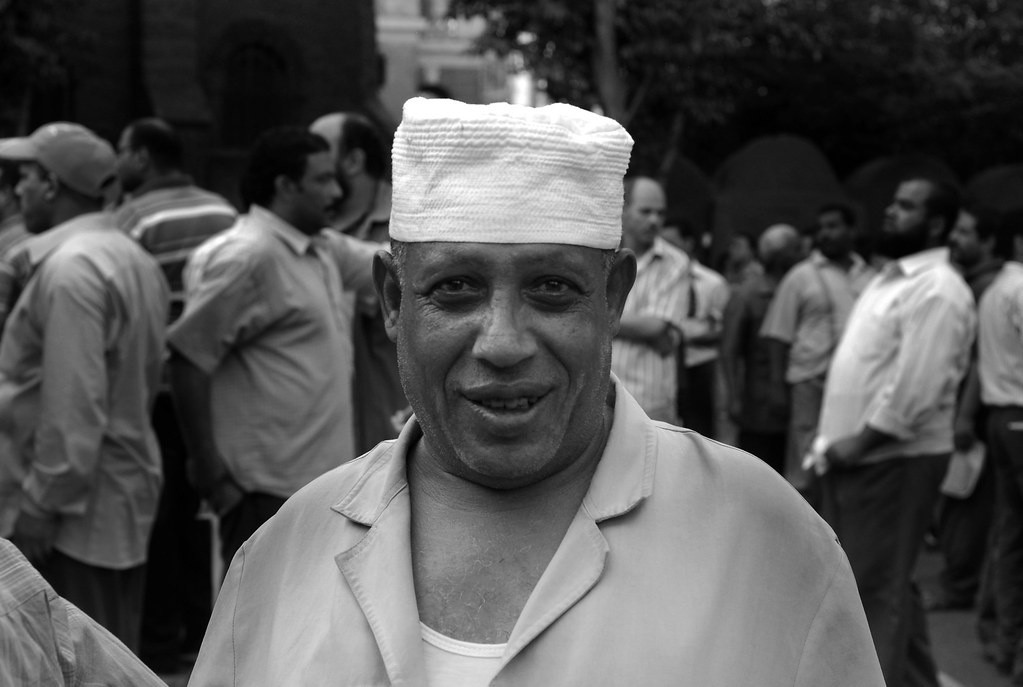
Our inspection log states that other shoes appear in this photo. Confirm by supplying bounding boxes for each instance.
[920,585,973,613]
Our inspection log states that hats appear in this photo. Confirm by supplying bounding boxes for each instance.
[0,121,120,202]
[388,95,634,250]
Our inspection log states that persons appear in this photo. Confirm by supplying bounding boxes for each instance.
[0,83,1023,687]
